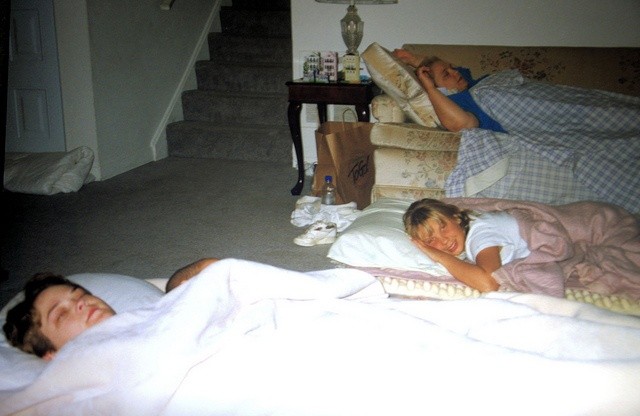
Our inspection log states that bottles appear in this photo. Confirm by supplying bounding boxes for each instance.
[321,176,336,205]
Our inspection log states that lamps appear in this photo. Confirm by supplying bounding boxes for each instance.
[316,1,397,55]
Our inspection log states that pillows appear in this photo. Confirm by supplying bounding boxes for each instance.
[361,40,441,129]
[0,272,165,392]
[328,198,467,277]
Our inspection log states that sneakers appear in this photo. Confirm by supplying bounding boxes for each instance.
[293,221,337,247]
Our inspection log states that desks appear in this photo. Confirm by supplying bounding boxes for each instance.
[287,77,381,196]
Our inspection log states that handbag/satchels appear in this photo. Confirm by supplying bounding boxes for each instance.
[308,108,375,210]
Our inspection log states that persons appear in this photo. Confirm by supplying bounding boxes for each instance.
[403,196,530,295]
[4,257,219,360]
[392,48,507,134]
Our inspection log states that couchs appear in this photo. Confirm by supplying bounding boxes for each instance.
[361,41,636,204]
